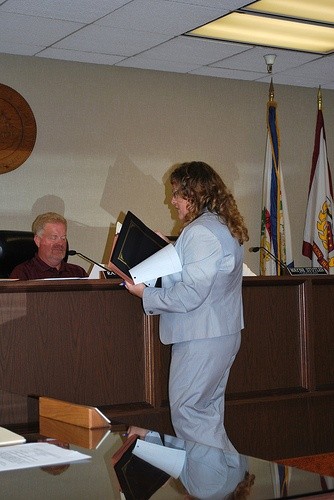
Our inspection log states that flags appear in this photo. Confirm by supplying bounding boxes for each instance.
[302,110,334,275]
[259,103,294,275]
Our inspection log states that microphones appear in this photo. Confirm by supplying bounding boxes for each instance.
[253,247,287,269]
[68,250,110,272]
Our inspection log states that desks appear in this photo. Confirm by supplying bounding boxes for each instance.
[0,414,334,500]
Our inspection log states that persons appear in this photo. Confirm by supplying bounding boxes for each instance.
[126,429,256,500]
[8,212,89,279]
[124,161,250,453]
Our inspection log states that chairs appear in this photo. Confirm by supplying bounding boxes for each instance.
[1,229,70,278]
